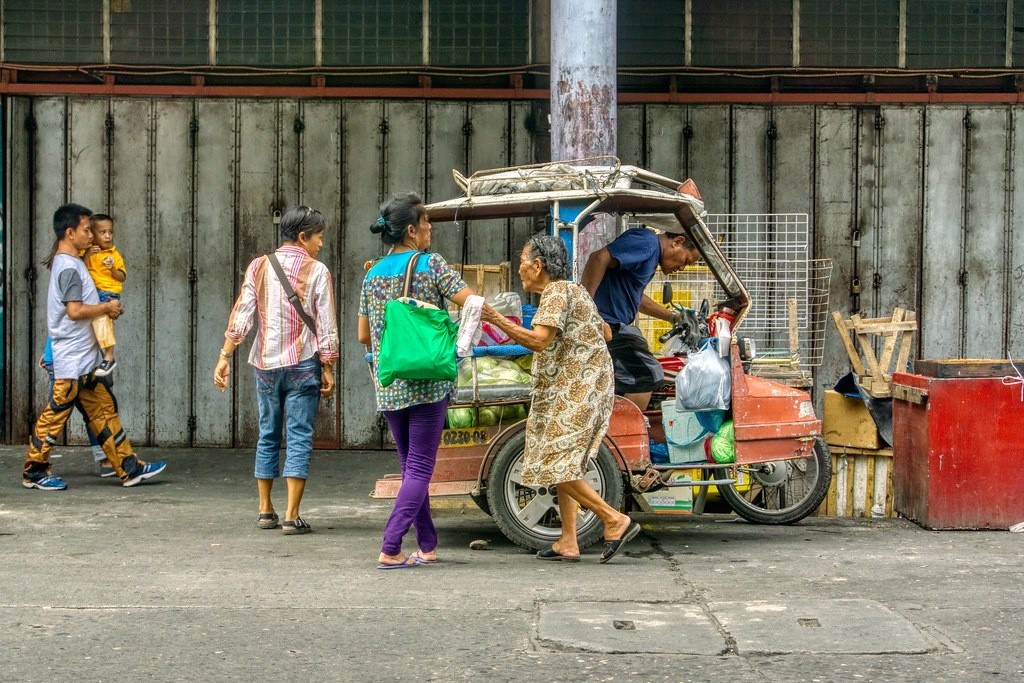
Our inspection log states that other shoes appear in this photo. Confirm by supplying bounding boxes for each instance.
[49,472,62,480]
[97,463,119,477]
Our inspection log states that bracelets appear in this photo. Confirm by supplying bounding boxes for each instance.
[221,349,232,358]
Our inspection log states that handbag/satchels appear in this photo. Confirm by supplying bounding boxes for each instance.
[441,290,525,349]
[661,338,735,464]
[376,252,460,389]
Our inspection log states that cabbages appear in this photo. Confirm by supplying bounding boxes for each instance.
[448,354,535,428]
[711,422,735,463]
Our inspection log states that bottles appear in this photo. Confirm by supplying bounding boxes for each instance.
[708,306,735,338]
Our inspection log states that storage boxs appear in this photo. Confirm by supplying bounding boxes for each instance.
[632,465,694,514]
[693,463,752,501]
[824,390,884,449]
[661,400,713,463]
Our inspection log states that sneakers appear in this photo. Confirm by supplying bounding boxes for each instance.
[93,358,117,377]
[21,474,67,491]
[119,460,167,487]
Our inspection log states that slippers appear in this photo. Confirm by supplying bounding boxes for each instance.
[534,544,582,563]
[409,549,438,563]
[376,557,420,569]
[597,521,642,564]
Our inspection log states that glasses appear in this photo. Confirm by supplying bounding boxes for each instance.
[297,205,316,233]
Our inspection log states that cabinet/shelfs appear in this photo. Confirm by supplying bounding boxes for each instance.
[892,371,1023,531]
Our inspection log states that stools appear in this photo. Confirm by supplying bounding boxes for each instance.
[835,304,917,403]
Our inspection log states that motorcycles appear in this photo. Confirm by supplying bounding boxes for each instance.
[368,160,838,561]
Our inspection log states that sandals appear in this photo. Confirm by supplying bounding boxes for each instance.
[280,516,312,535]
[254,507,280,529]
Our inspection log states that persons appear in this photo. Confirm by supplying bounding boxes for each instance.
[214,206,339,535]
[359,192,476,569]
[461,234,640,564]
[84,214,126,376]
[23,203,166,490]
[581,229,701,393]
